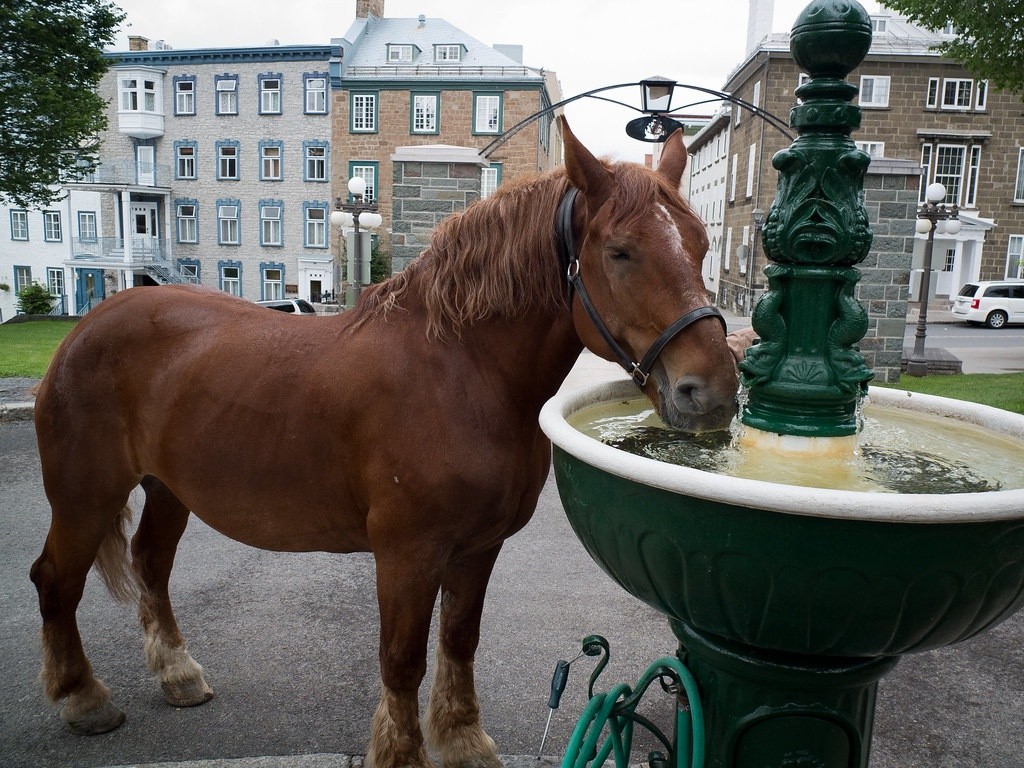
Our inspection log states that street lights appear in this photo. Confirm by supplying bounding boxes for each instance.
[903,183,962,379]
[329,175,382,305]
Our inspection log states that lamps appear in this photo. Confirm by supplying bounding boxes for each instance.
[750,209,764,230]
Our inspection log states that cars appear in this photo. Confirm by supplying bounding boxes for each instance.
[950,279,1024,330]
[252,296,316,316]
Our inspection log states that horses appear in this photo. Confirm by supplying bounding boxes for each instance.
[28,113,740,768]
